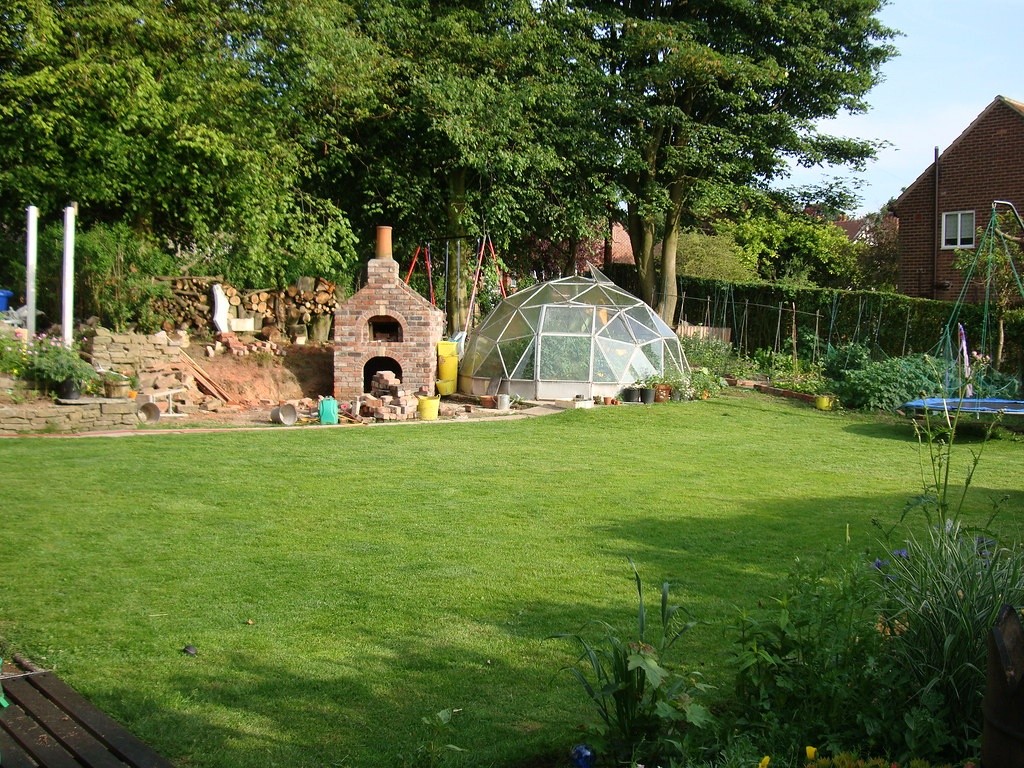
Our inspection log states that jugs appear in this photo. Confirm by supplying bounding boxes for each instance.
[492,393,517,410]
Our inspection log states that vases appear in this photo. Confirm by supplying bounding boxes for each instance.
[479,395,498,409]
[603,397,611,405]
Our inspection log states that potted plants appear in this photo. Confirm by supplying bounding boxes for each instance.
[814,392,839,411]
[614,394,622,405]
[624,379,642,402]
[640,370,721,404]
[100,372,138,399]
[40,351,97,399]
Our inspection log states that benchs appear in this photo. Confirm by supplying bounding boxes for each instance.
[137,388,188,417]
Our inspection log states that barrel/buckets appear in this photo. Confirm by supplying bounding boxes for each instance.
[418,394,441,421]
[435,378,454,395]
